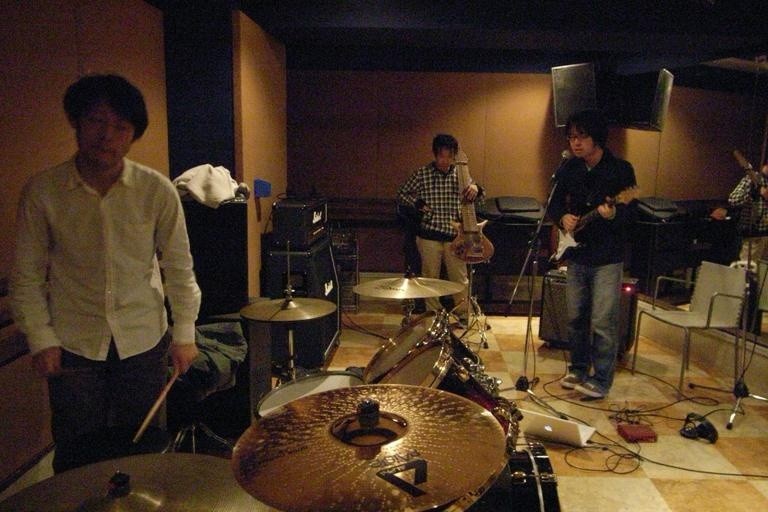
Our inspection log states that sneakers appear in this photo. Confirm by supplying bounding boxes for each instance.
[560,373,584,388]
[575,381,603,398]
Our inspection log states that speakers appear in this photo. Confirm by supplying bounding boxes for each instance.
[263,237,342,367]
[539,269,640,353]
[181,201,249,316]
[614,68,674,132]
[551,61,617,129]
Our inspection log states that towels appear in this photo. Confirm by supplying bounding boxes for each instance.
[171,164,239,210]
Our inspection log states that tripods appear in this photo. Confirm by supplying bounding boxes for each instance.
[499,179,569,422]
[687,191,768,430]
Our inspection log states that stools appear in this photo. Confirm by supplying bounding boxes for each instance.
[162,361,237,458]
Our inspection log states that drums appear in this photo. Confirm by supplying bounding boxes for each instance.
[257,370,365,416]
[468,441,561,511]
[363,307,485,397]
[375,339,520,449]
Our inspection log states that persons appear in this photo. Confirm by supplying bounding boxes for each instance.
[10,70,205,475]
[727,156,767,268]
[396,132,486,326]
[547,110,640,401]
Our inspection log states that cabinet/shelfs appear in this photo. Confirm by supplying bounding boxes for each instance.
[331,236,360,314]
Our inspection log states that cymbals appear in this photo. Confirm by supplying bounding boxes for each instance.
[231,383,507,511]
[0,452,276,512]
[351,265,465,300]
[239,297,337,323]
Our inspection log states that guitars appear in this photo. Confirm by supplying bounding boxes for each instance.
[549,178,648,266]
[730,147,768,202]
[449,144,495,264]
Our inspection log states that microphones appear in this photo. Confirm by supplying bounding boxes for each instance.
[733,148,763,191]
[550,149,572,181]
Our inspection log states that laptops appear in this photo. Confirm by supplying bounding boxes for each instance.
[517,407,597,448]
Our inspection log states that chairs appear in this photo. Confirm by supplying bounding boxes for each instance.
[631,261,746,401]
